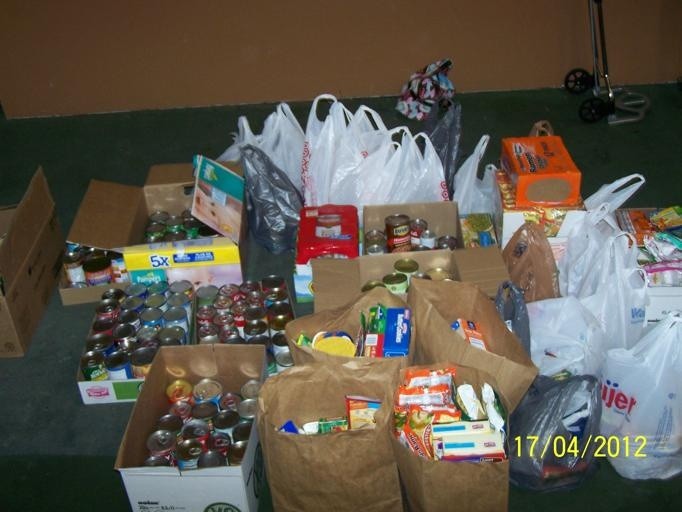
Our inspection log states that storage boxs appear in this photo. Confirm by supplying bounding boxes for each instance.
[500,136,580,206]
[60,253,129,305]
[309,246,510,312]
[295,205,359,302]
[65,155,248,286]
[1,167,65,356]
[115,345,266,511]
[364,202,458,256]
[77,280,192,404]
[495,170,582,248]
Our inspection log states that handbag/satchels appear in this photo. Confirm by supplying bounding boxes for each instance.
[395,58,455,122]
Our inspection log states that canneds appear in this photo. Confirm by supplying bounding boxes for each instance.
[144,378,258,467]
[361,212,456,296]
[62,210,222,289]
[81,278,294,381]
